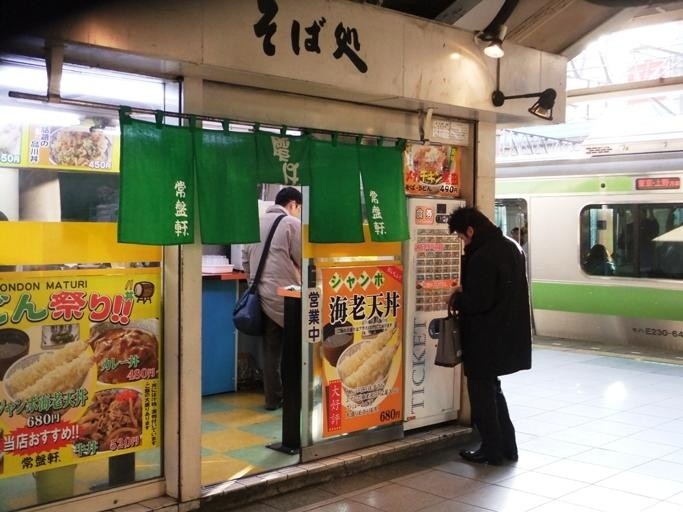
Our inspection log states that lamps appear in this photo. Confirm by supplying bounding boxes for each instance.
[474,25,556,121]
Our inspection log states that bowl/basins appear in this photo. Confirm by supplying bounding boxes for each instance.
[407,155,456,194]
[321,320,393,407]
[0,328,91,420]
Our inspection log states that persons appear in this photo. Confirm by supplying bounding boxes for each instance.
[586,243,613,275]
[509,224,527,253]
[444,204,534,468]
[663,203,683,262]
[618,202,661,271]
[240,186,303,414]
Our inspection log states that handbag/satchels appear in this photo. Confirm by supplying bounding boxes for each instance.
[432,293,463,368]
[233,285,267,338]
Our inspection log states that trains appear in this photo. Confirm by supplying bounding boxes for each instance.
[493,148,683,355]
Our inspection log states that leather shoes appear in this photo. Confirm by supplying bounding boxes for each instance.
[459,448,505,467]
[505,450,518,461]
